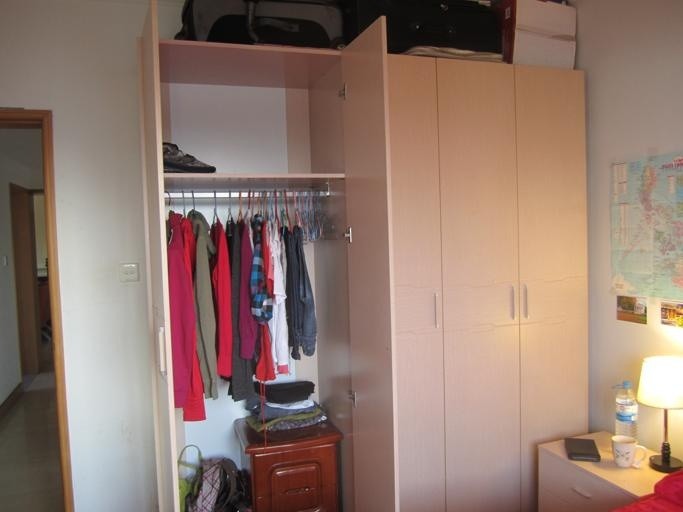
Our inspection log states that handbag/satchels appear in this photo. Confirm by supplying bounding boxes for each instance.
[177,444,252,511]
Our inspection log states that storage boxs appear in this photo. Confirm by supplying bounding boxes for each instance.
[492,0,577,69]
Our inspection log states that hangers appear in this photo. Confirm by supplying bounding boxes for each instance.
[162,180,338,240]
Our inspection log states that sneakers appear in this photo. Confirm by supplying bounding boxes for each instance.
[163,142,216,173]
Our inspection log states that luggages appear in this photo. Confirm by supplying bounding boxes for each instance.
[174,0,349,50]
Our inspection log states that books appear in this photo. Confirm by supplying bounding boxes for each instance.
[566,438,601,462]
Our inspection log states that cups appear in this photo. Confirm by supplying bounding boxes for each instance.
[611,435,648,468]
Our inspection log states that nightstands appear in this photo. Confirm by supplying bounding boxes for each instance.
[535,429,672,512]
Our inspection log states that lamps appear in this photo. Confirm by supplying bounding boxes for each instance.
[636,354,683,473]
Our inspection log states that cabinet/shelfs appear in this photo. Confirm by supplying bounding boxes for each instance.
[234,416,343,512]
[136,0,593,511]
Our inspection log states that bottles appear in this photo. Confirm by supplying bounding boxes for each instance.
[614,380,639,439]
